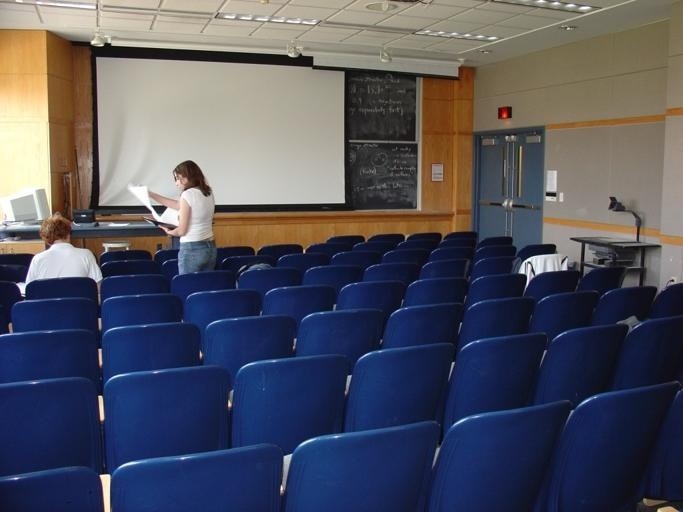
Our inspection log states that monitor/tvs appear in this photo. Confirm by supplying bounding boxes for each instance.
[2,187,49,226]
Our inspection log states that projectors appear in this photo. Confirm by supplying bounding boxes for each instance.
[72,210,94,223]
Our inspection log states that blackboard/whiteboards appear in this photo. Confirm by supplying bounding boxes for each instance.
[345,69,419,209]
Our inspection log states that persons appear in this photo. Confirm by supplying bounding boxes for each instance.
[23,214,103,305]
[139,160,216,274]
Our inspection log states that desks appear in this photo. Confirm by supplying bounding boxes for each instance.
[569,236,661,285]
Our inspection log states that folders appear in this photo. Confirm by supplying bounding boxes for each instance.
[143,217,177,229]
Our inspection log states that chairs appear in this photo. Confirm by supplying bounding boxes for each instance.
[533,291,597,331]
[99,322,201,378]
[528,271,579,294]
[338,280,405,307]
[281,420,435,510]
[348,343,455,431]
[403,277,465,303]
[0,231,556,260]
[467,272,526,295]
[0,467,108,512]
[181,290,259,323]
[462,296,533,340]
[470,256,512,272]
[301,266,360,288]
[645,385,683,504]
[168,271,236,294]
[263,286,333,316]
[448,331,546,425]
[612,316,682,389]
[295,308,385,354]
[649,282,680,314]
[429,399,569,511]
[360,263,417,280]
[421,259,467,274]
[237,269,297,288]
[100,274,166,295]
[0,378,101,468]
[100,294,181,328]
[99,259,157,274]
[600,285,655,322]
[383,303,462,347]
[0,281,20,328]
[105,364,228,471]
[534,323,628,405]
[11,298,100,331]
[578,266,626,290]
[549,381,677,511]
[0,328,101,380]
[201,313,294,364]
[26,277,97,299]
[111,443,281,511]
[228,354,348,454]
